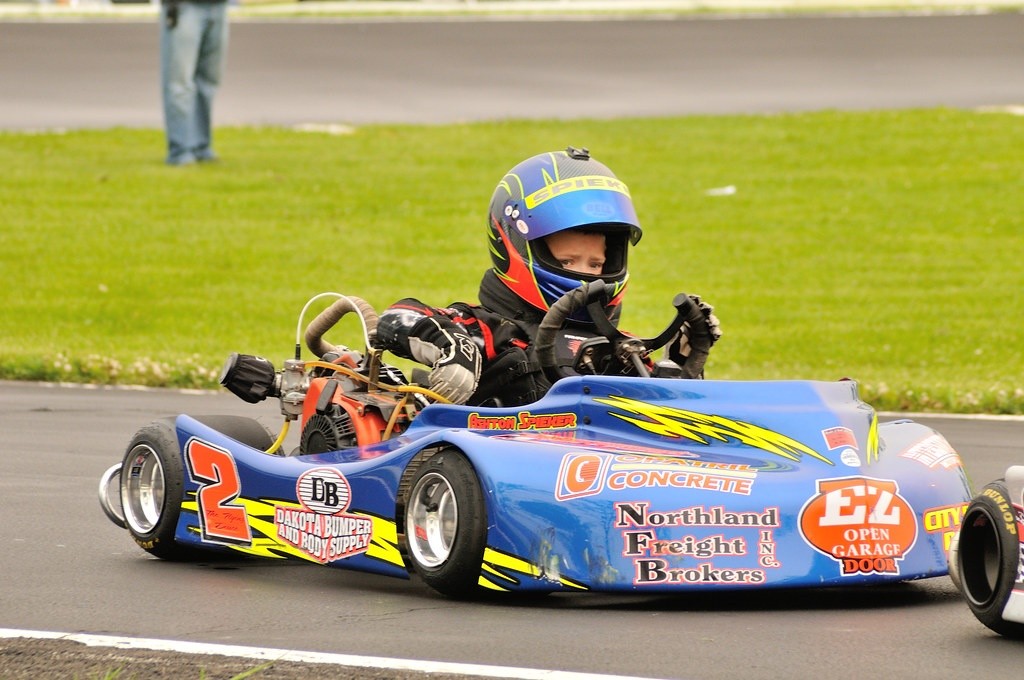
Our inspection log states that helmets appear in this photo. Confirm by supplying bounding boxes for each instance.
[478,145,643,334]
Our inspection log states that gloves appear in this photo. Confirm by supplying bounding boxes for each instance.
[649,295,724,380]
[407,316,483,407]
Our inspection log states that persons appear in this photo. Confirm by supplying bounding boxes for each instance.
[378,146,725,410]
[156,0,228,167]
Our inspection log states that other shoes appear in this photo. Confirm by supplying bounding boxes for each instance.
[167,152,195,166]
[196,148,217,162]
